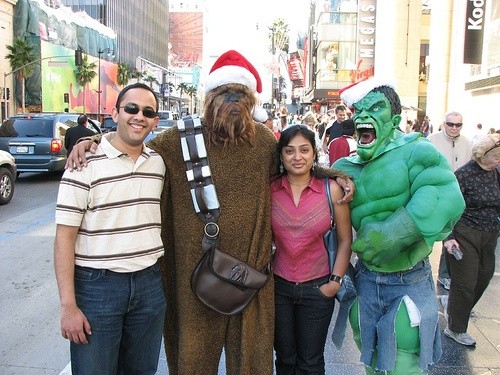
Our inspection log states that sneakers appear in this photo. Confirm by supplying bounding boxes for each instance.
[440,295,450,323]
[444,326,476,346]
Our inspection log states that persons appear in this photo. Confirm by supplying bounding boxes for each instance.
[269,126,352,375]
[64,51,355,375]
[53,83,167,375]
[65,114,95,158]
[403,115,500,146]
[329,84,466,375]
[444,136,500,346]
[264,103,359,168]
[425,111,472,291]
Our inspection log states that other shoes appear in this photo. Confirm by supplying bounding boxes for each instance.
[437,277,451,289]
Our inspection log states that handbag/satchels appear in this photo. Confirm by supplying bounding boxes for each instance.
[189,245,270,317]
[322,177,358,301]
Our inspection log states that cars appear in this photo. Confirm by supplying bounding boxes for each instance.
[101,115,118,133]
[0,113,103,179]
[142,111,180,143]
[0,149,17,206]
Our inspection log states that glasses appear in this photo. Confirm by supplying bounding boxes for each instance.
[484,140,500,157]
[280,124,308,136]
[446,121,463,127]
[118,103,159,119]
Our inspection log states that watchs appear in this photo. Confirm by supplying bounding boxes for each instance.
[329,274,343,286]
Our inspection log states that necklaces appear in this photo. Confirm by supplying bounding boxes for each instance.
[287,175,312,186]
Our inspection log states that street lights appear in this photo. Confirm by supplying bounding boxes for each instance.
[92,47,116,122]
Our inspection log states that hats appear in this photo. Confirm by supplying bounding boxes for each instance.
[337,76,381,114]
[254,108,278,122]
[205,50,262,95]
